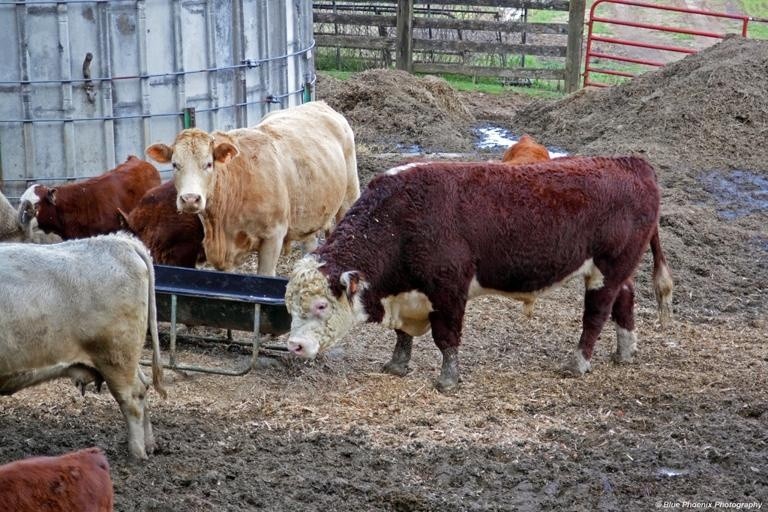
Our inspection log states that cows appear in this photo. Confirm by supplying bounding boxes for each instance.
[283,132,675,394]
[0,98,362,280]
[0,231,167,462]
[0,447,114,512]
